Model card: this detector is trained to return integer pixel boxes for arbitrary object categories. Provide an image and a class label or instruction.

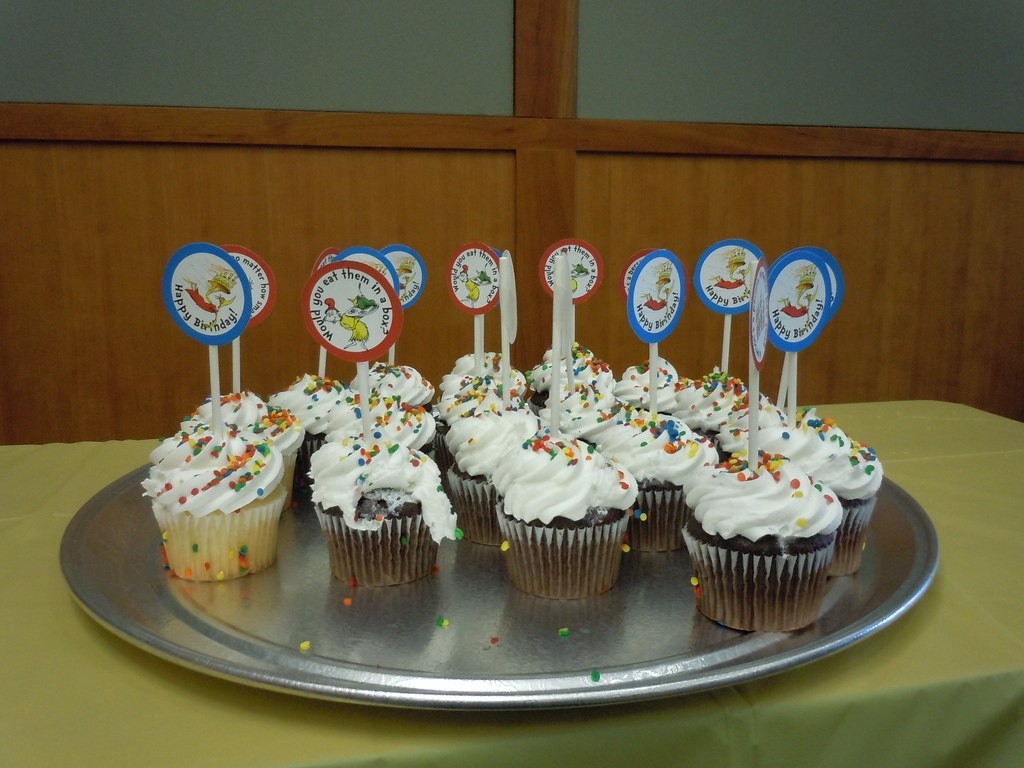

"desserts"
[139,339,884,631]
